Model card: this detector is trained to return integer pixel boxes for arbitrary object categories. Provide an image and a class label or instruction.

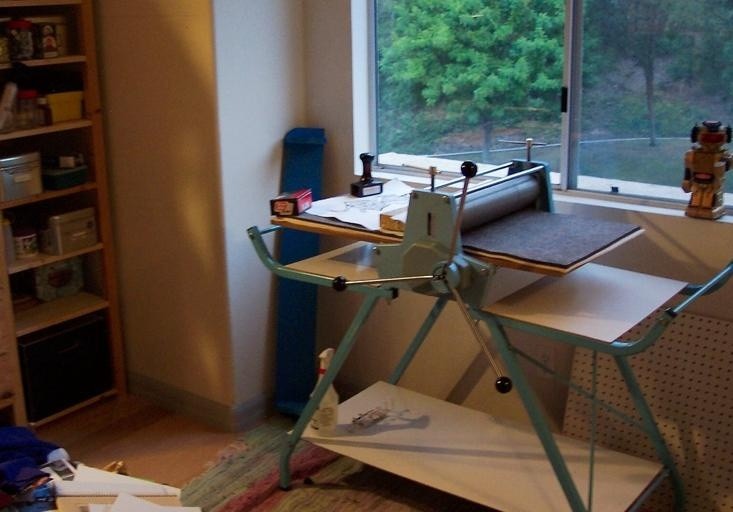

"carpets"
[178,410,490,512]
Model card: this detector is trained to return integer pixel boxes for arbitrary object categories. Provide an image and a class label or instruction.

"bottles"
[2,219,16,267]
[35,98,51,126]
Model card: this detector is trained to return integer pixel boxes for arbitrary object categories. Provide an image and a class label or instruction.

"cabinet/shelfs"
[0,0,127,429]
